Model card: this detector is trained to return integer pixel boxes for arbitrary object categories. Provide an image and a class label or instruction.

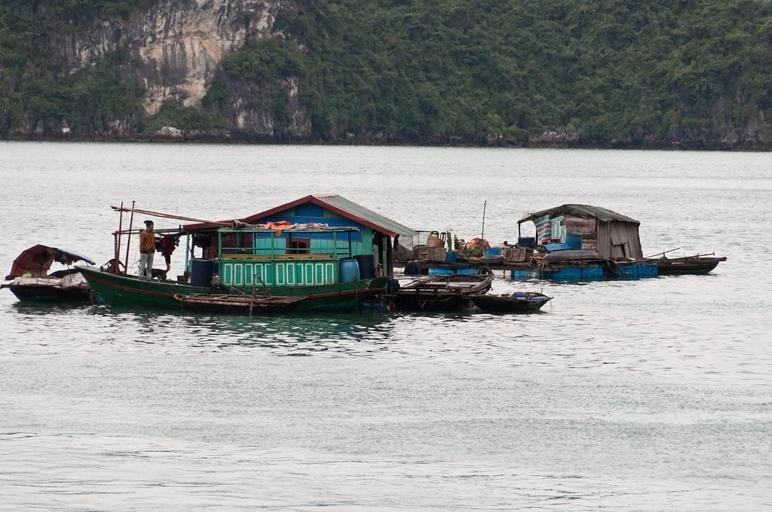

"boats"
[0,244,100,302]
[75,194,552,315]
[401,273,495,297]
[392,203,728,284]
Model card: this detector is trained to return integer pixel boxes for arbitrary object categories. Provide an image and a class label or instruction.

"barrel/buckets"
[518,237,535,248]
[191,258,214,286]
[341,258,361,284]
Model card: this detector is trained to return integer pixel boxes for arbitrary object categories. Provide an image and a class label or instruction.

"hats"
[144,220,153,226]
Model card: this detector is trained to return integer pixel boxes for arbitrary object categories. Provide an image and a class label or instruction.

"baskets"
[426,231,446,248]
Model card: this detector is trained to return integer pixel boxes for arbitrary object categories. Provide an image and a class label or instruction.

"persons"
[394,235,399,261]
[139,220,155,280]
